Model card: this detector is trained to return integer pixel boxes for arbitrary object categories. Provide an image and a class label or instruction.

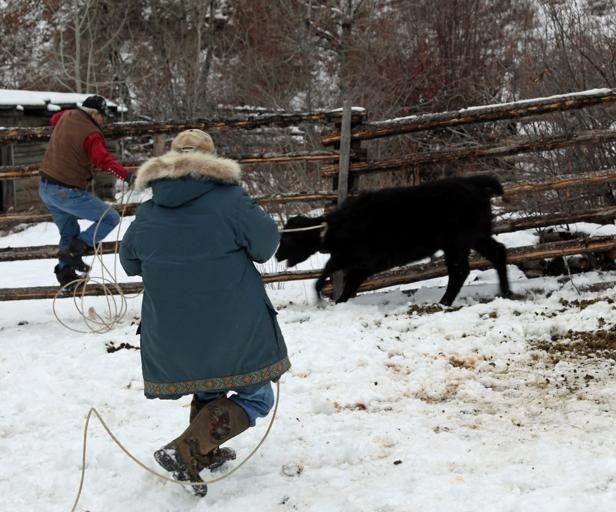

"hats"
[170,129,215,155]
[81,95,113,118]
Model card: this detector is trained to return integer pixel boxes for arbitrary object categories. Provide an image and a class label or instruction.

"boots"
[153,395,249,497]
[54,234,89,292]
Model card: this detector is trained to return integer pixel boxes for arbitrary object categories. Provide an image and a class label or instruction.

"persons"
[40,95,134,290]
[119,128,292,497]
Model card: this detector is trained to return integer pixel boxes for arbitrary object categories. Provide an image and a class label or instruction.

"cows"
[274,173,513,307]
